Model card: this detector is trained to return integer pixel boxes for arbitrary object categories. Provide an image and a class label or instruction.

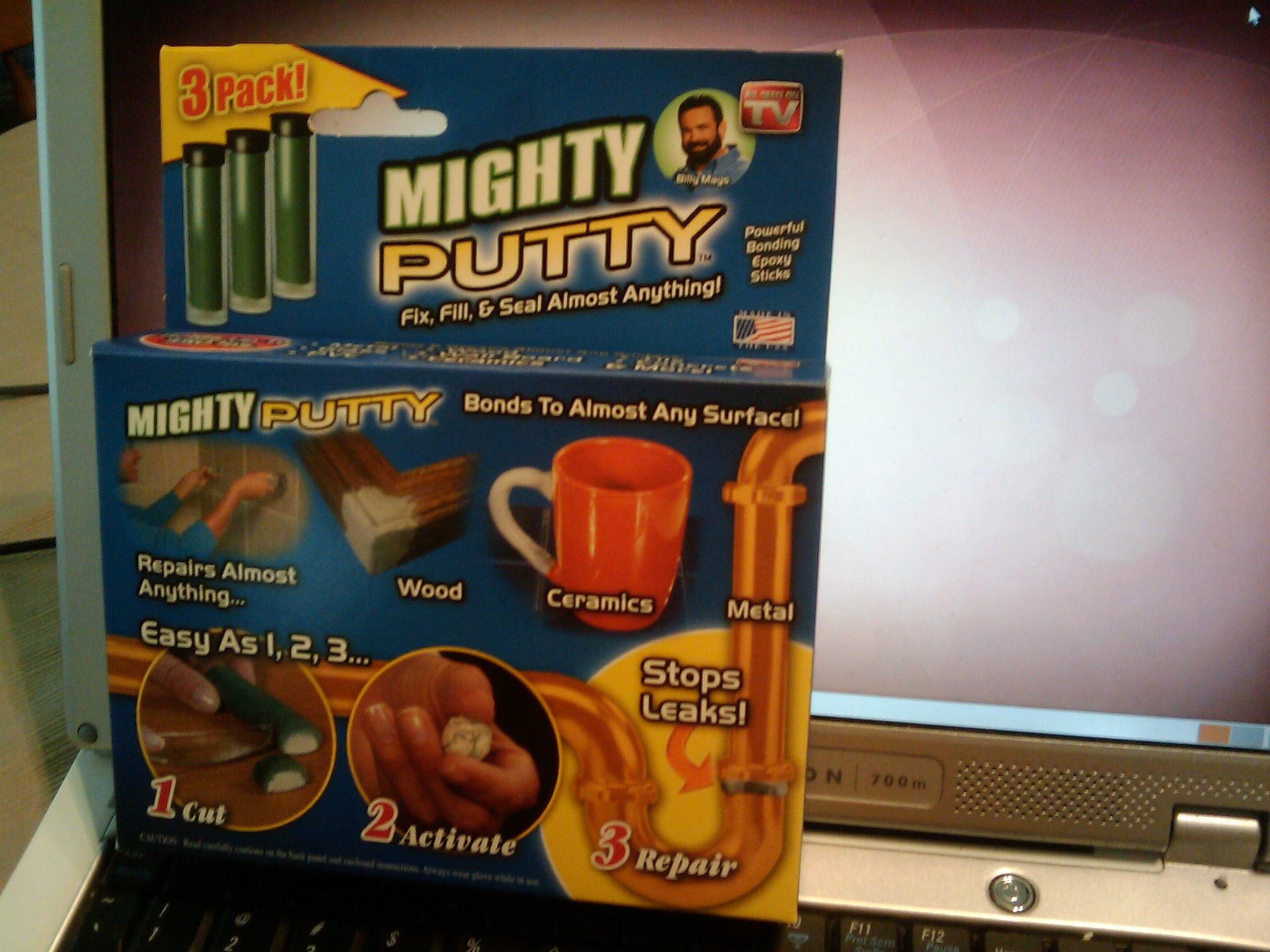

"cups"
[490,437,693,631]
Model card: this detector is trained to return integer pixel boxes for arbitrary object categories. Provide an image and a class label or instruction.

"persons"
[670,95,749,182]
[353,653,538,831]
[141,645,260,757]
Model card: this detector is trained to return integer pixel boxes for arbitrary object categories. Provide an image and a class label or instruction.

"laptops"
[0,0,1270,952]
